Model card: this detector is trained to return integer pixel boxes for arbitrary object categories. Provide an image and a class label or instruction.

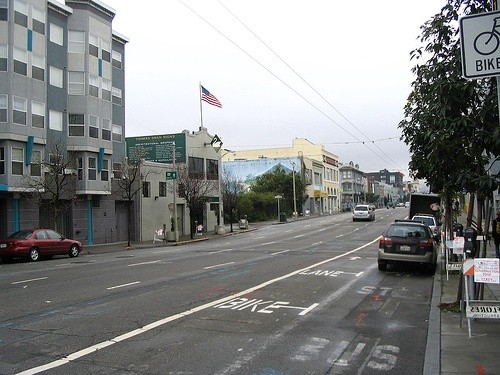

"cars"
[352,204,376,223]
[411,214,439,244]
[0,227,84,263]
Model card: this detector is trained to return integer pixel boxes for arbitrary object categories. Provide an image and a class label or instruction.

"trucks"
[409,191,441,226]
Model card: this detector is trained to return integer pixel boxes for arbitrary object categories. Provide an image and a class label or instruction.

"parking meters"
[462,227,477,318]
[453,223,464,275]
[454,200,459,223]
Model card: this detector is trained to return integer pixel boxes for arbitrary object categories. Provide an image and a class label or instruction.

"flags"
[201,86,222,108]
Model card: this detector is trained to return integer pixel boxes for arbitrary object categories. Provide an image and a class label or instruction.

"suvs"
[377,219,438,276]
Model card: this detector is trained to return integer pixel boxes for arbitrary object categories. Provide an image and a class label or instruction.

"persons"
[491,211,500,258]
[455,199,459,210]
